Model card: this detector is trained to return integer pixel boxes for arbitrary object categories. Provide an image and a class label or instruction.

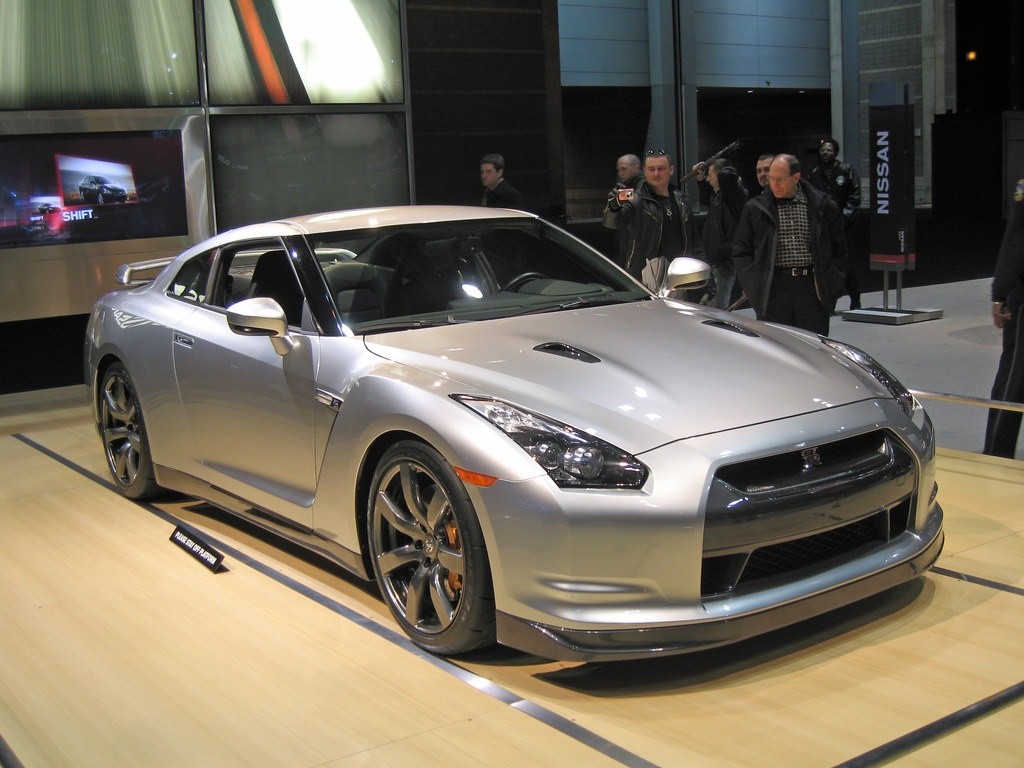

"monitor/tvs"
[0,129,188,249]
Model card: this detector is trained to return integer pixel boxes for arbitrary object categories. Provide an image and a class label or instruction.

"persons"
[400,244,467,316]
[478,153,525,293]
[983,177,1024,461]
[601,138,863,338]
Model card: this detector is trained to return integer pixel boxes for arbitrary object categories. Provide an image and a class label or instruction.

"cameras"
[618,188,634,200]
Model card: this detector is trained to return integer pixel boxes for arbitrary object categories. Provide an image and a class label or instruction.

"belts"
[773,266,816,277]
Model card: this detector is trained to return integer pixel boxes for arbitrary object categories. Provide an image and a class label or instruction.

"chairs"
[246,232,575,331]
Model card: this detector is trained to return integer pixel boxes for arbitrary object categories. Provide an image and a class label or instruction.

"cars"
[80,176,129,204]
[86,204,945,655]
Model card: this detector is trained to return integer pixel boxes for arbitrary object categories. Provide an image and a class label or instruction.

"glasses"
[645,150,668,158]
